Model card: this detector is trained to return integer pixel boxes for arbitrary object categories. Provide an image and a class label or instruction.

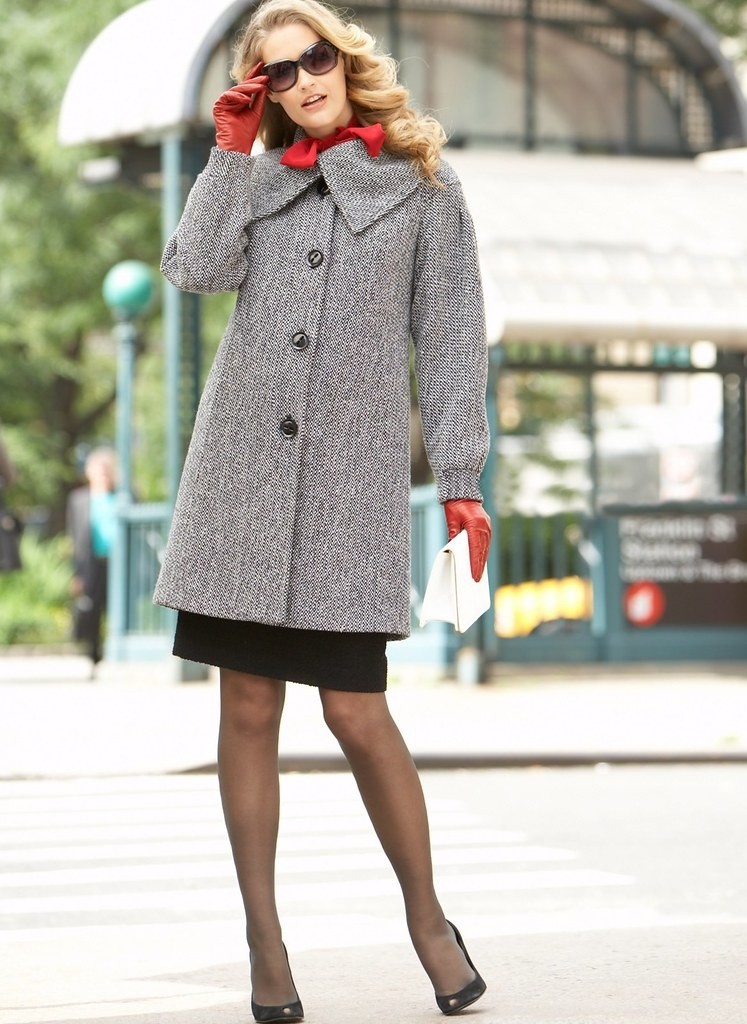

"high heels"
[250,940,304,1023]
[434,920,486,1016]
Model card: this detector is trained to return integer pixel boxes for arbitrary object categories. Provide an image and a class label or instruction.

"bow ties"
[280,113,387,169]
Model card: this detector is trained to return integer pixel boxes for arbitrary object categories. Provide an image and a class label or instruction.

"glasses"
[259,41,339,93]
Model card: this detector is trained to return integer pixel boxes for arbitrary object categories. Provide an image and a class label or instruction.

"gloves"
[213,62,269,155]
[444,499,492,582]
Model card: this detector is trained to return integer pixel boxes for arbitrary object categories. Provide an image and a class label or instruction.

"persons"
[152,0,492,1024]
[68,447,134,679]
